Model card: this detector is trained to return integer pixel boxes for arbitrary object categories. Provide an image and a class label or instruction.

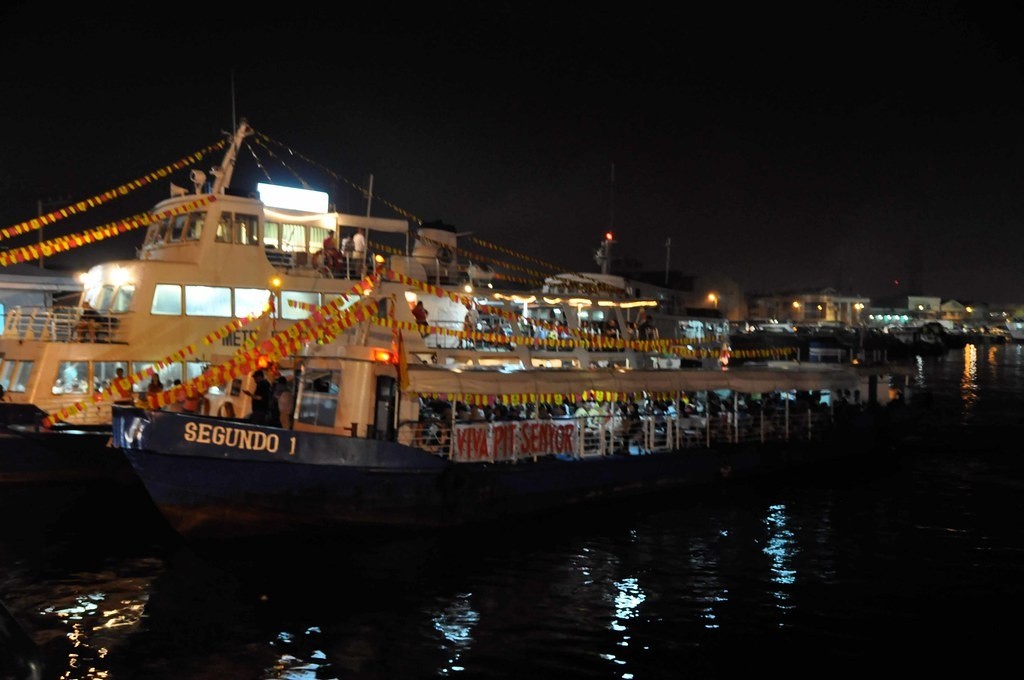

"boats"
[0,117,971,574]
[115,352,871,535]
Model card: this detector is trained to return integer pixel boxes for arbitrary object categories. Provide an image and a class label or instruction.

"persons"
[183,398,200,414]
[273,377,293,428]
[77,302,99,343]
[411,301,430,338]
[462,313,652,353]
[324,226,367,269]
[148,375,163,395]
[242,370,273,426]
[112,369,124,382]
[420,391,856,462]
